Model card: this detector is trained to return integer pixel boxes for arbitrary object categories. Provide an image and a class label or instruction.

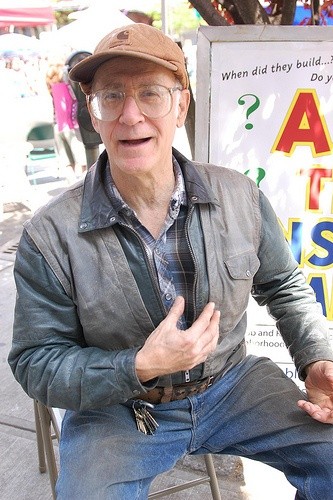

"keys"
[132,399,159,437]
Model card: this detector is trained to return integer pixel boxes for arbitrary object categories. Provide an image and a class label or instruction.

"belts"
[134,344,246,405]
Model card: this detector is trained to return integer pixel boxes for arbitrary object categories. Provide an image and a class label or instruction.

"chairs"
[34,399,221,500]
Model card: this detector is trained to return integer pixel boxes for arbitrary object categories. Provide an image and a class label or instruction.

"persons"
[47,64,86,180]
[8,23,332,500]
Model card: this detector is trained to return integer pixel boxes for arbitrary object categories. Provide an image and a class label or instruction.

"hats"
[68,23,189,89]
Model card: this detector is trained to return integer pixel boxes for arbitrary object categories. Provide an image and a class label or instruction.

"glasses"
[85,84,184,122]
